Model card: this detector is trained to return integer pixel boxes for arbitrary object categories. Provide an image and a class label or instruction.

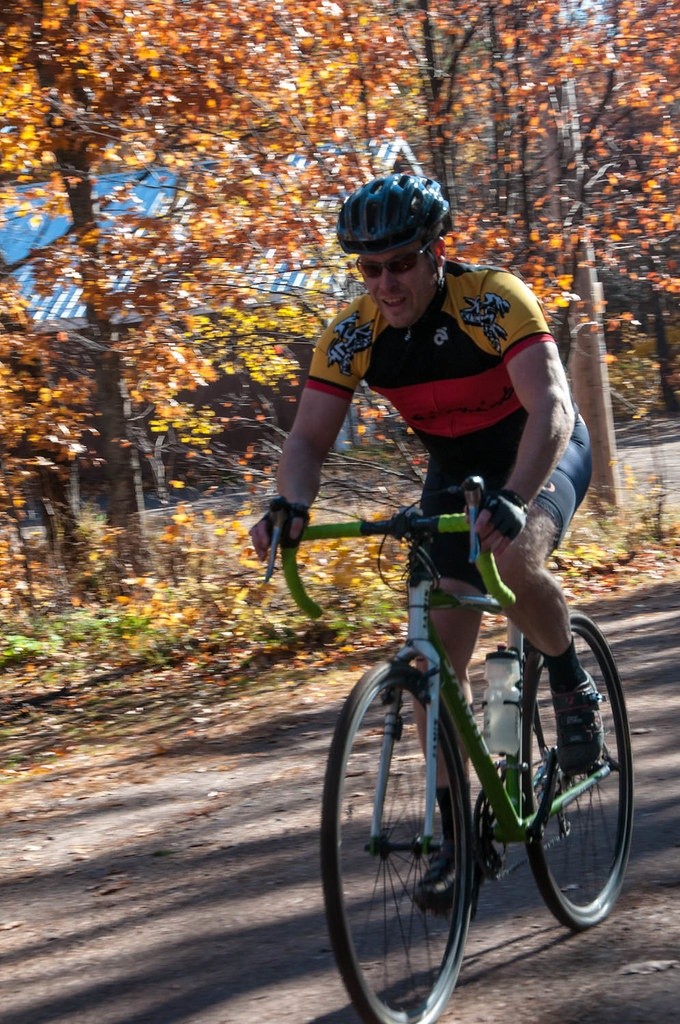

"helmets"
[335,175,451,255]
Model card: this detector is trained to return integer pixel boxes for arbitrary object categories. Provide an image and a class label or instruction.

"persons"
[251,174,604,905]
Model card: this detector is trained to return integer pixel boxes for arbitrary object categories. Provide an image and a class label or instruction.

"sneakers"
[412,834,457,907]
[552,667,608,776]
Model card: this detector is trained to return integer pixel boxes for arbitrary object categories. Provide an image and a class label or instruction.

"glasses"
[355,235,440,279]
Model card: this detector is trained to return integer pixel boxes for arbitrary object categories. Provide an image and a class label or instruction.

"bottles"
[482,643,520,755]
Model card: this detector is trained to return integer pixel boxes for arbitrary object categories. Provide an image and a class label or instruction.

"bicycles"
[265,474,633,1024]
